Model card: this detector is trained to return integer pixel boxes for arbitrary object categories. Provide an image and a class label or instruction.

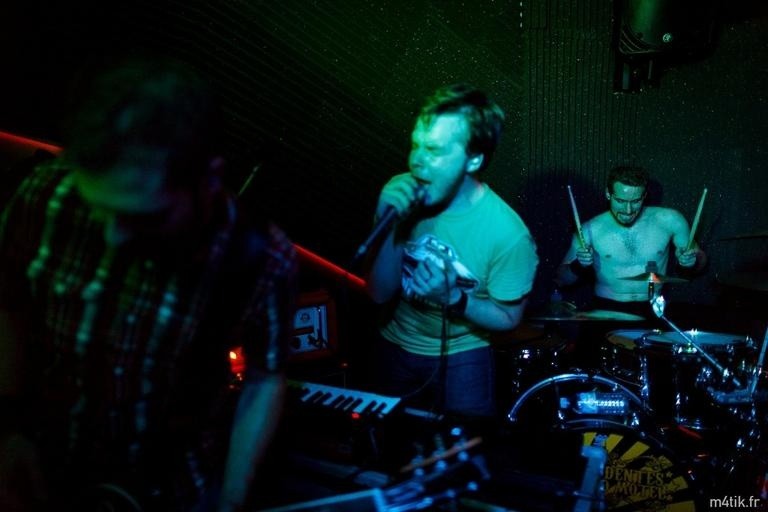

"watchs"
[443,294,468,327]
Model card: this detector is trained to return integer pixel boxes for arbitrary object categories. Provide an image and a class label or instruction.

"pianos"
[222,366,403,465]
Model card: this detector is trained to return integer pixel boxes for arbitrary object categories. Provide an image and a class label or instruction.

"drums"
[671,342,752,430]
[601,329,654,388]
[499,368,713,511]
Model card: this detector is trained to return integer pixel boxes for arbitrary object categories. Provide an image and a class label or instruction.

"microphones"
[350,182,422,263]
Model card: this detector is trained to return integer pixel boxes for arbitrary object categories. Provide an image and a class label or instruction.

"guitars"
[0,426,494,512]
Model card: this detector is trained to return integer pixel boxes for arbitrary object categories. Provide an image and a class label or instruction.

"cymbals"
[625,273,690,284]
[646,329,748,346]
[524,309,646,323]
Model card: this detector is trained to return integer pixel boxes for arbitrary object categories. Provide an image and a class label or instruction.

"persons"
[351,91,541,432]
[560,174,698,316]
